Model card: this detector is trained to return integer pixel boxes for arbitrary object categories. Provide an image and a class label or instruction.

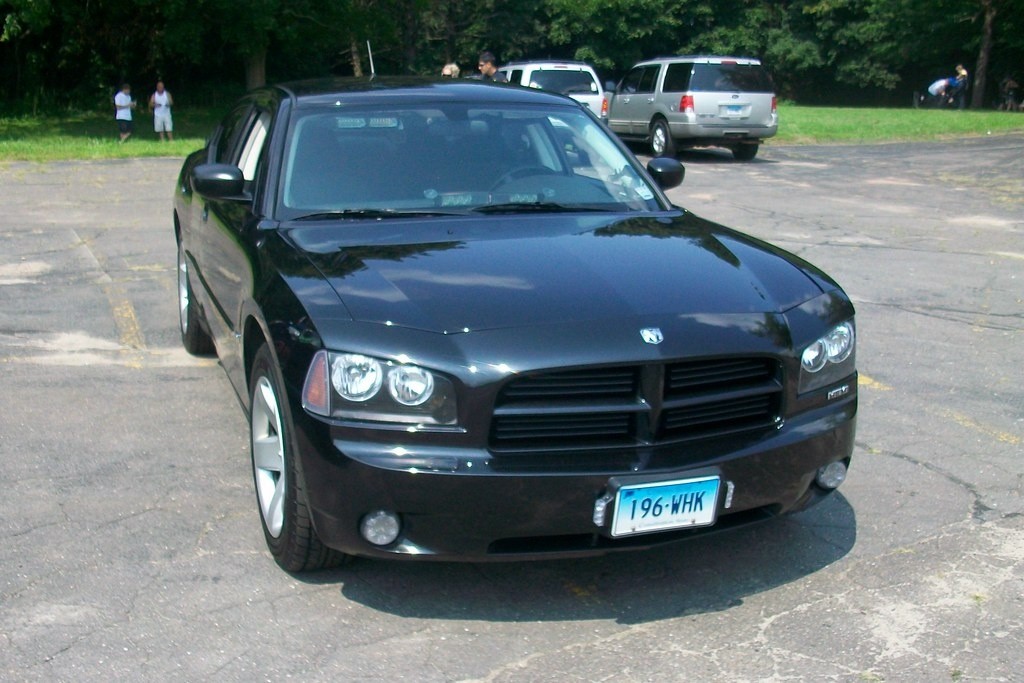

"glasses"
[478,64,487,67]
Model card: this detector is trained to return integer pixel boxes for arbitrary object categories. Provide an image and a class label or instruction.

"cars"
[496,60,608,122]
[170,72,859,574]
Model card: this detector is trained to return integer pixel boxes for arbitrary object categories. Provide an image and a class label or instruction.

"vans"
[608,54,778,161]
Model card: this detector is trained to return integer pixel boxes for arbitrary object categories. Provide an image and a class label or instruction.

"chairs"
[288,130,348,205]
[440,132,514,192]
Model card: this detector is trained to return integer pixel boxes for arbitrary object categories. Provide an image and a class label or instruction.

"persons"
[441,63,461,79]
[920,64,968,109]
[114,84,136,145]
[479,52,509,83]
[150,81,173,143]
[998,76,1024,112]
